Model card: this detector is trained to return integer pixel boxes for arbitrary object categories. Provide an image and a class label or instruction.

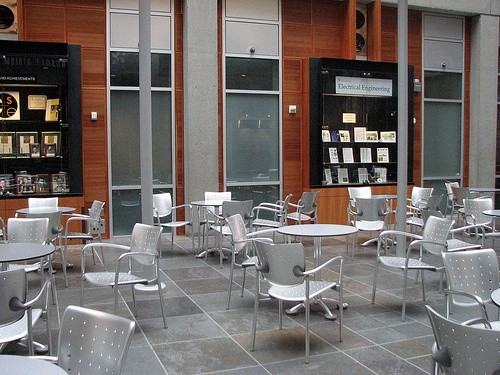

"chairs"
[348,181,500,375]
[0,191,344,375]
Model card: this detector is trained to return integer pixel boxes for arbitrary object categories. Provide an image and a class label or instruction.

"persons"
[0,146,65,196]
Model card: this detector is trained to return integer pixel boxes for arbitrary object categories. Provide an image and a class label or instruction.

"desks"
[467,187,500,239]
[0,201,359,375]
[355,193,398,247]
[481,210,500,218]
